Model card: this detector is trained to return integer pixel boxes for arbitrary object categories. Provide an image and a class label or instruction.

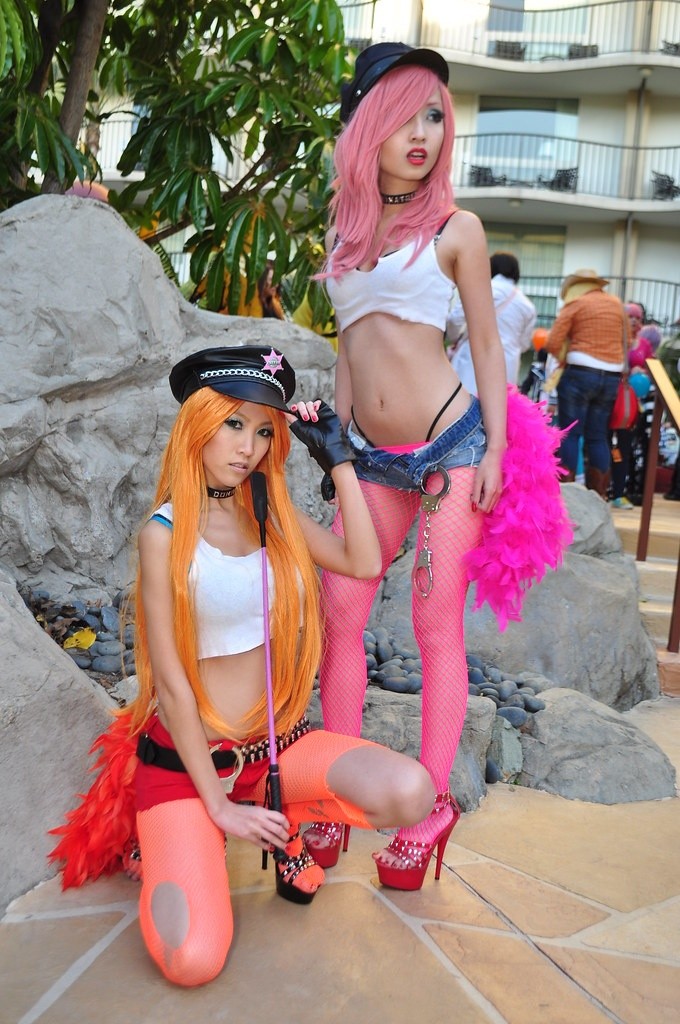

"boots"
[588,466,610,501]
[560,468,575,482]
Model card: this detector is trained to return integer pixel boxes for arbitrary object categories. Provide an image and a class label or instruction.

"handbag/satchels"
[610,379,638,429]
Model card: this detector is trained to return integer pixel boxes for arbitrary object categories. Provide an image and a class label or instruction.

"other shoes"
[612,497,634,510]
[664,490,680,501]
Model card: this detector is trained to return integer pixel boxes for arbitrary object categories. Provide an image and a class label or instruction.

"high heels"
[117,851,142,863]
[261,835,321,905]
[301,821,351,867]
[375,788,461,890]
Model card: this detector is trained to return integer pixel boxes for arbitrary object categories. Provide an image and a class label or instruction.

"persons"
[118,345,437,987]
[319,41,510,884]
[450,251,680,510]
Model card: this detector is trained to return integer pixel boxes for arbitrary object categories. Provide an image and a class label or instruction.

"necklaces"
[380,189,419,205]
[207,487,236,499]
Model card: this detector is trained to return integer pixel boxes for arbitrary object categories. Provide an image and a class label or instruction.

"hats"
[561,268,610,299]
[169,345,300,418]
[340,41,449,121]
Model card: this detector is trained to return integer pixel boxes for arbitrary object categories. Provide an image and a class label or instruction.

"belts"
[568,363,622,376]
[137,714,311,773]
